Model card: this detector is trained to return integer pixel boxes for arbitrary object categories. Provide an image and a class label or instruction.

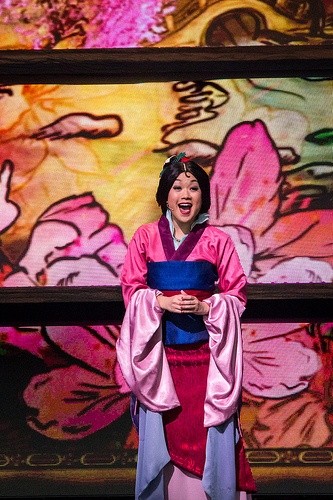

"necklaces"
[173,235,181,243]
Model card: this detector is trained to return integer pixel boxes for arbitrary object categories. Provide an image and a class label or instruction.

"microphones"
[166,201,173,211]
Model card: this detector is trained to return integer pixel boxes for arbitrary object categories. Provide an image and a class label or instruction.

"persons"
[113,155,261,500]
